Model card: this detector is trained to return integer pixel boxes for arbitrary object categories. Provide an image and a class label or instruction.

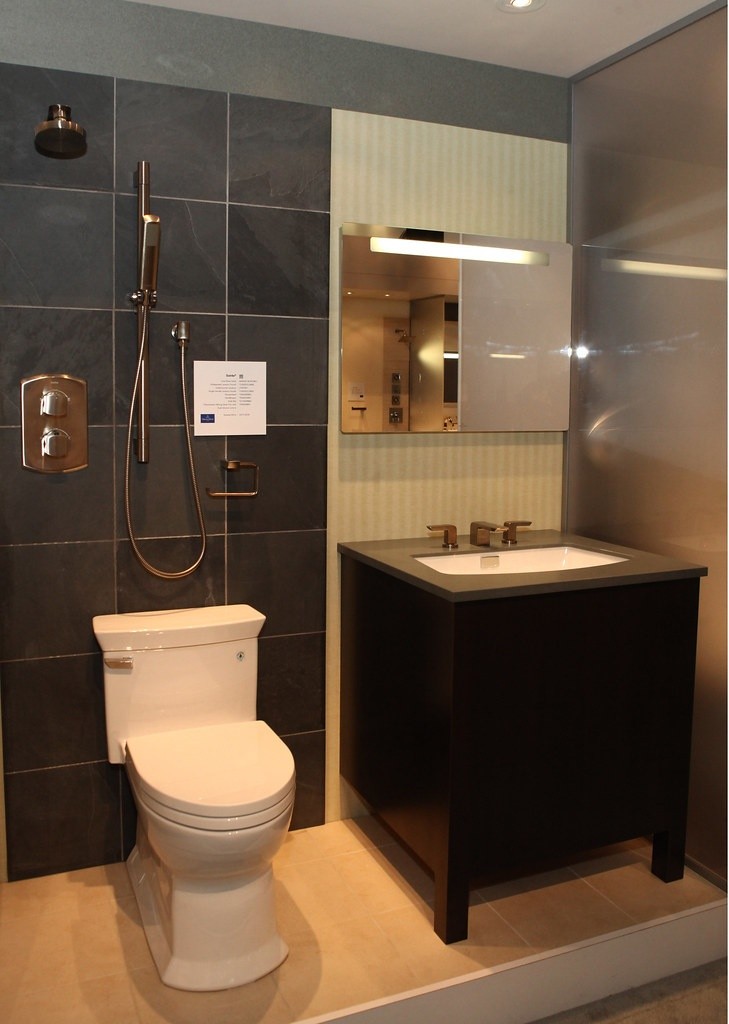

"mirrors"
[338,221,458,440]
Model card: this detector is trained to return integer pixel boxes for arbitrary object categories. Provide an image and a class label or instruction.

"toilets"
[91,600,298,994]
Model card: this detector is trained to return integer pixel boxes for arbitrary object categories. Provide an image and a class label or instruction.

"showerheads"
[135,212,163,309]
[31,102,89,161]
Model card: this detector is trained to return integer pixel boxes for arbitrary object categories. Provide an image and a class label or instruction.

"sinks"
[409,539,638,578]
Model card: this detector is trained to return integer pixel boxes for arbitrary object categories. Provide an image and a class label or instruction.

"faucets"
[469,520,509,548]
